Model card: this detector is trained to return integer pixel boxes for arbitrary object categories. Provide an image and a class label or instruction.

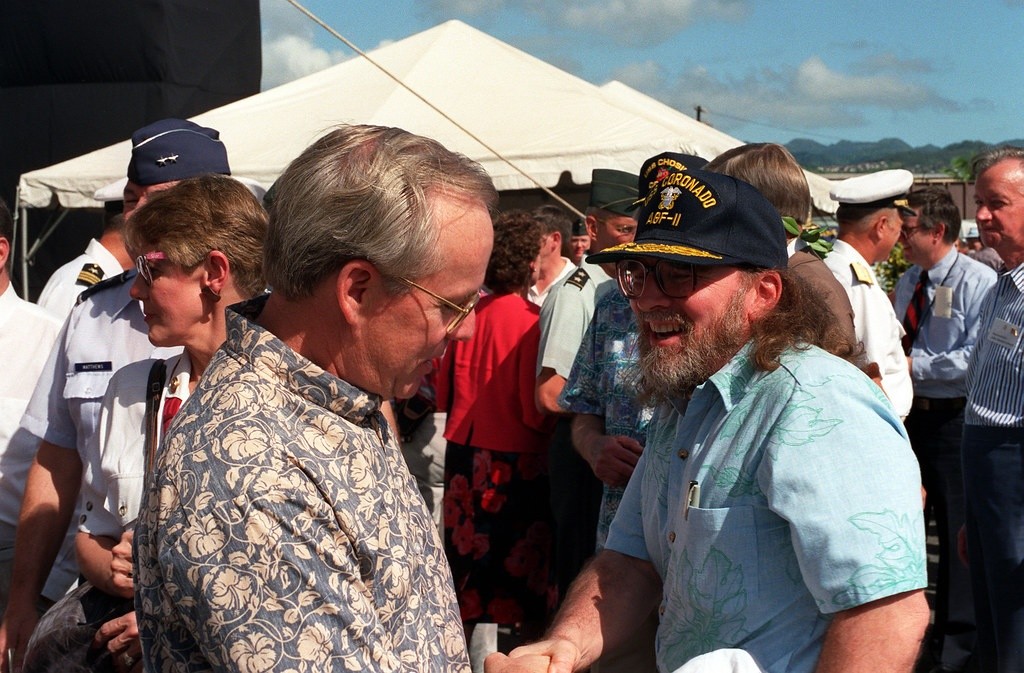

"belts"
[913,396,968,410]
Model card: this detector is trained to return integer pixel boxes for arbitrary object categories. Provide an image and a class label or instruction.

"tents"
[18,19,840,302]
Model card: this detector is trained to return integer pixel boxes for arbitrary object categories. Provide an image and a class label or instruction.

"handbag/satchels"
[21,577,120,673]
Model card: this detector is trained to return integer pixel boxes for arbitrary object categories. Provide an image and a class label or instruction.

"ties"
[900,269,930,351]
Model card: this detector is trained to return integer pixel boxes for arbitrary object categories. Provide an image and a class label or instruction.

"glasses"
[329,252,482,336]
[135,248,172,287]
[615,258,697,300]
[595,214,639,236]
[900,223,939,237]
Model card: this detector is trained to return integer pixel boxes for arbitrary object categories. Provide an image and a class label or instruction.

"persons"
[382,169,656,652]
[823,169,914,422]
[964,226,1004,272]
[0,199,79,603]
[36,174,136,316]
[509,171,931,673]
[891,186,998,673]
[75,172,270,673]
[958,144,1024,673]
[139,124,551,673]
[0,119,233,673]
[701,142,857,365]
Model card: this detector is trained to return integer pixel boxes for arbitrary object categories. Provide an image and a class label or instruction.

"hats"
[589,167,645,220]
[636,152,710,208]
[127,119,231,186]
[584,169,788,278]
[828,168,918,220]
